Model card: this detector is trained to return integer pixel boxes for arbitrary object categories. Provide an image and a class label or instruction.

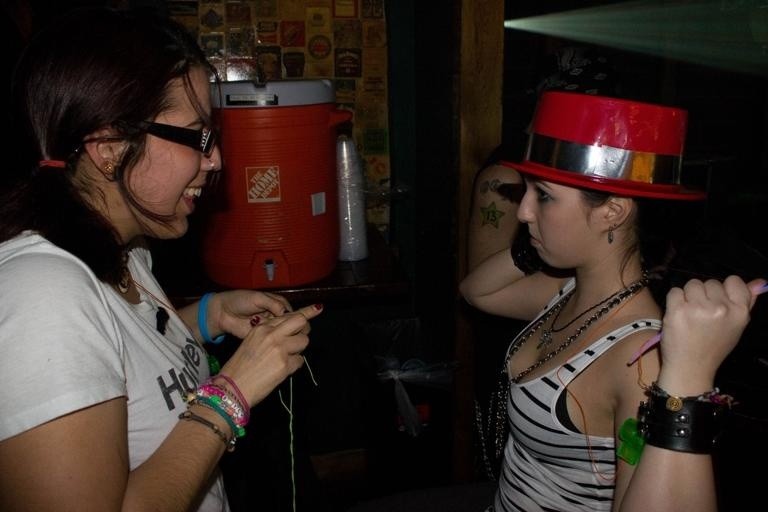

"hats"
[493,87,710,202]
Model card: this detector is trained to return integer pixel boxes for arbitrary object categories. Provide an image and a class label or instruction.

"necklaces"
[484,270,654,461]
[118,252,132,293]
[535,289,623,350]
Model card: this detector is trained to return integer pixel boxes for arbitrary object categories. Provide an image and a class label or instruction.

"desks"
[155,223,411,507]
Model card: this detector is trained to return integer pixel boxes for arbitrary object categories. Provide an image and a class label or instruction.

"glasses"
[114,116,220,159]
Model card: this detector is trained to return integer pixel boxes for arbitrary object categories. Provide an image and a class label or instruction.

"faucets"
[262,255,278,282]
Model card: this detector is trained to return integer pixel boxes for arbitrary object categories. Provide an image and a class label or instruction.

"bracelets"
[176,371,253,453]
[511,236,541,276]
[198,290,227,346]
[636,381,737,455]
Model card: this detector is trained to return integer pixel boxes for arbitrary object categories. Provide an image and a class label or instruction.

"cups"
[336,134,370,263]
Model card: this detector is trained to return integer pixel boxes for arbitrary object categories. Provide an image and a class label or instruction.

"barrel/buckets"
[193,76,340,290]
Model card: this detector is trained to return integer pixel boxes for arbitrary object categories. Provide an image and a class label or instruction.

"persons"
[0,9,326,512]
[464,140,525,483]
[455,91,767,511]
